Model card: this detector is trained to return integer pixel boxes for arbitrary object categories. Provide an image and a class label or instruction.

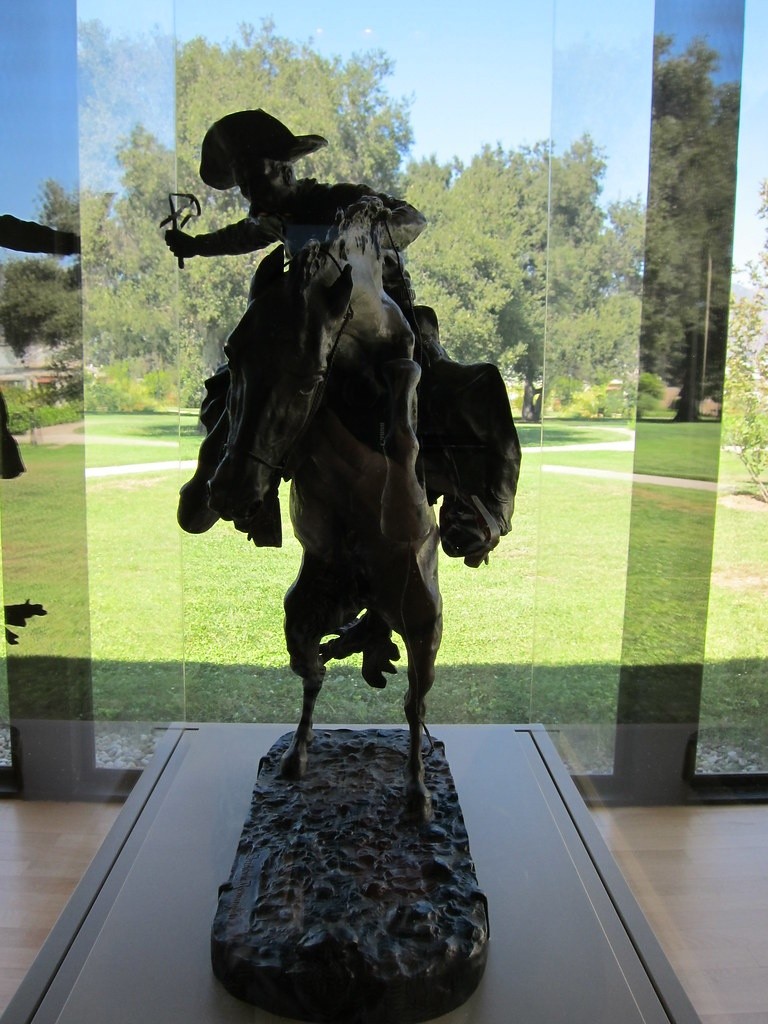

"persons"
[159,109,515,557]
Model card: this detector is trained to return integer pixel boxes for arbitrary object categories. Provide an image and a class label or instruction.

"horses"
[204,244,444,824]
